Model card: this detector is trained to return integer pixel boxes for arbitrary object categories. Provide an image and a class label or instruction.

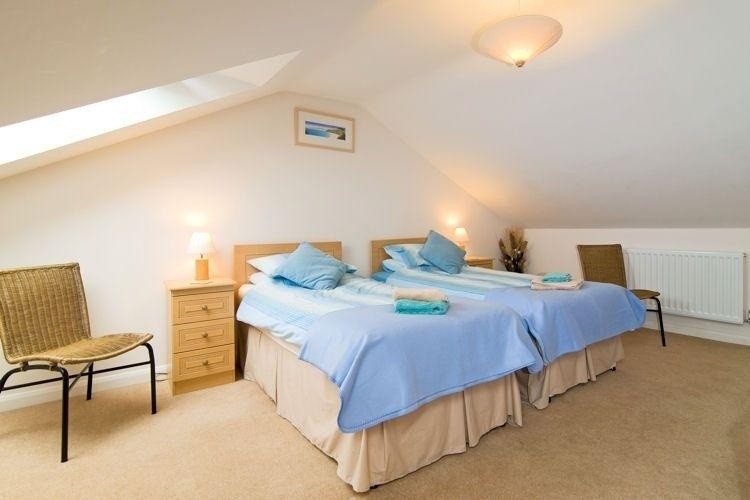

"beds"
[228,237,548,494]
[369,233,651,414]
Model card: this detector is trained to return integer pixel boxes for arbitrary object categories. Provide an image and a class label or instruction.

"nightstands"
[460,253,496,271]
[163,272,240,400]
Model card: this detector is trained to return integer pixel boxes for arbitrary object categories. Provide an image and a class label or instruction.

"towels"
[541,270,573,284]
[392,283,451,300]
[395,298,454,317]
[528,273,587,294]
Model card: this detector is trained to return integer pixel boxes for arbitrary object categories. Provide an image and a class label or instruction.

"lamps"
[470,12,567,73]
[186,228,216,284]
[450,222,471,256]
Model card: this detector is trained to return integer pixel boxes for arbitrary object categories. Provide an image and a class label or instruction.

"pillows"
[243,228,470,292]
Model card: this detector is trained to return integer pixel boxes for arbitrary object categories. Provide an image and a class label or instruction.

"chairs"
[574,242,670,351]
[1,259,160,468]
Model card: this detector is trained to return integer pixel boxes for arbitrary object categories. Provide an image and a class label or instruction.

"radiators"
[614,244,750,328]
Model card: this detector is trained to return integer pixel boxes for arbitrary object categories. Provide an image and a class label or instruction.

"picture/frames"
[292,104,358,157]
